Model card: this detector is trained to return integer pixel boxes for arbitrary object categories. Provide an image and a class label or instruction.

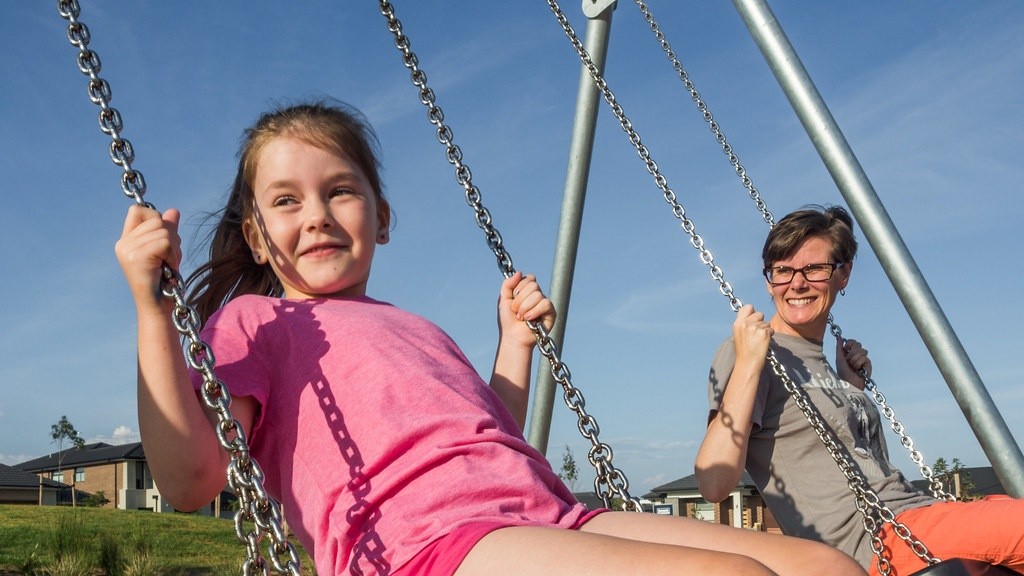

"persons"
[696,205,1024,576]
[115,102,869,576]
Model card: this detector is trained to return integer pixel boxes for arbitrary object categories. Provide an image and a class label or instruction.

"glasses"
[763,262,844,284]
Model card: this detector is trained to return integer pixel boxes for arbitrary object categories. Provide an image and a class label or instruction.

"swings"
[542,0,1024,576]
[52,0,648,576]
